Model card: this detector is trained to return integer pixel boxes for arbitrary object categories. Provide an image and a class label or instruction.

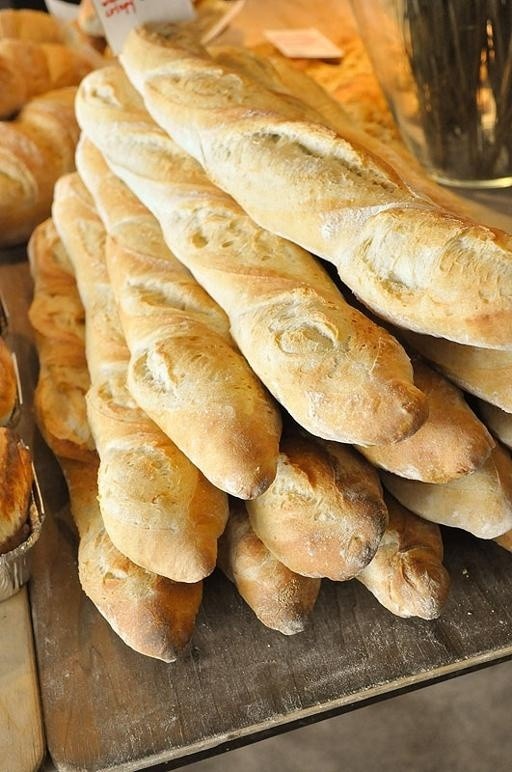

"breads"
[1,0,512,663]
